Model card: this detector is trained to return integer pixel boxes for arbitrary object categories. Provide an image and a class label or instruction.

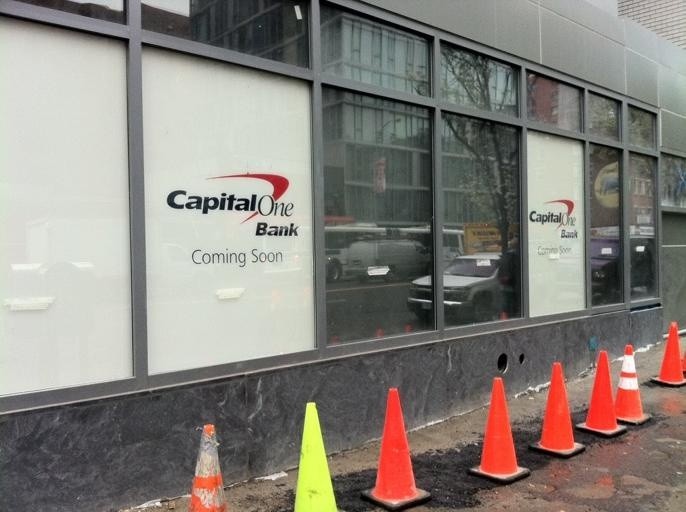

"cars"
[405,251,520,323]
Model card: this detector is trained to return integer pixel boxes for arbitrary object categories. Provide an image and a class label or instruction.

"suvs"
[590,235,656,305]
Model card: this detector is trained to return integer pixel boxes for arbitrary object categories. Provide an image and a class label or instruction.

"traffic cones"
[574,351,627,440]
[188,424,227,512]
[330,335,338,345]
[293,401,339,512]
[650,322,686,388]
[499,312,508,320]
[615,344,652,426]
[360,387,432,512]
[465,377,530,485]
[376,329,384,337]
[403,324,413,333]
[528,362,585,460]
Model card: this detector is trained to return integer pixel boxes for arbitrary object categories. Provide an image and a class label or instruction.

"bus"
[324,223,465,283]
